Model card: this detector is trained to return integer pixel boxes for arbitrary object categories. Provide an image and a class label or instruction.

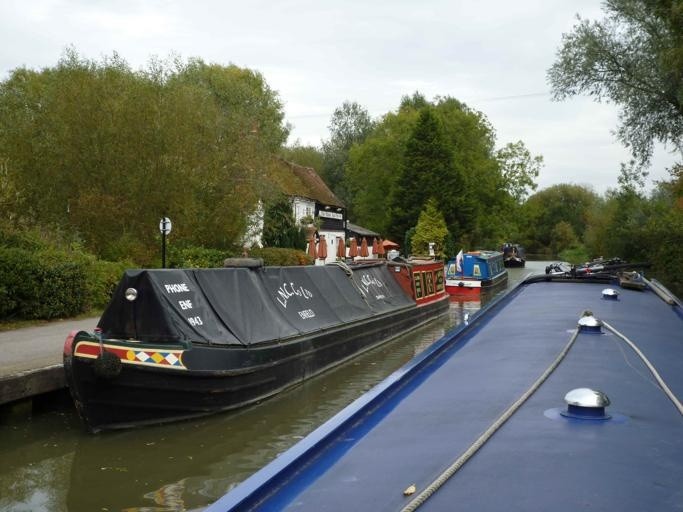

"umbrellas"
[347,239,358,262]
[334,239,345,261]
[380,239,399,249]
[377,238,386,259]
[316,234,328,265]
[306,237,318,262]
[358,238,369,260]
[371,238,378,259]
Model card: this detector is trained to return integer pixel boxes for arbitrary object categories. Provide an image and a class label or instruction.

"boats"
[503,243,525,267]
[445,250,508,295]
[196,258,683,507]
[64,258,450,439]
